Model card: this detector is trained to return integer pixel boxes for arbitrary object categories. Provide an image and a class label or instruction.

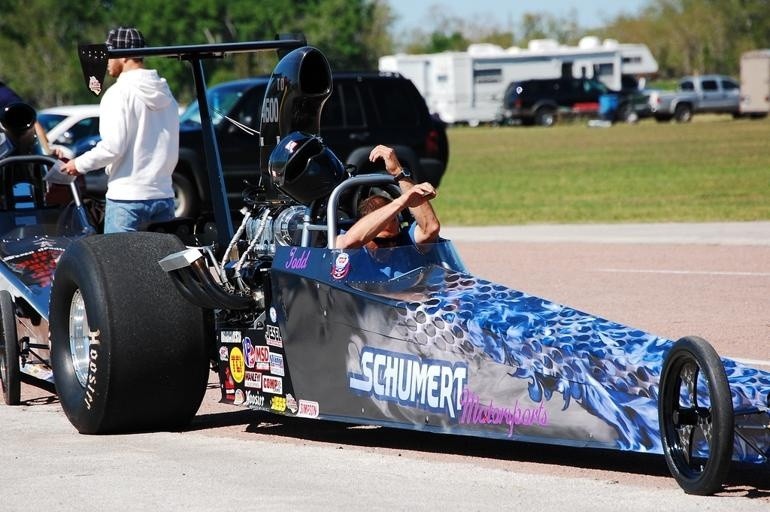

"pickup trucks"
[646,75,741,124]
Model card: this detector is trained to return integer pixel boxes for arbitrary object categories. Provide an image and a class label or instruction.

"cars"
[598,88,657,124]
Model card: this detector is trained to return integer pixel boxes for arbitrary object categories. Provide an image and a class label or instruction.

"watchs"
[394,168,411,180]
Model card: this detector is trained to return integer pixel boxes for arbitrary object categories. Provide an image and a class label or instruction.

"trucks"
[739,50,770,114]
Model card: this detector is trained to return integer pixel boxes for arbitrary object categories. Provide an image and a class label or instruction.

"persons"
[60,24,180,235]
[336,143,441,280]
[0,82,49,153]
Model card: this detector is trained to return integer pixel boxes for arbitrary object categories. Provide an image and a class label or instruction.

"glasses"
[372,224,406,247]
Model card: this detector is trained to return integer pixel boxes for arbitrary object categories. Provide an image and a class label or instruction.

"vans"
[499,77,620,127]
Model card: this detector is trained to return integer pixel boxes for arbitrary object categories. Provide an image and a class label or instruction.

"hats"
[105,26,146,49]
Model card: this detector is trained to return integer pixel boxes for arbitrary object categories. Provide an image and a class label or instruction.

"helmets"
[0,103,37,134]
[267,130,347,204]
